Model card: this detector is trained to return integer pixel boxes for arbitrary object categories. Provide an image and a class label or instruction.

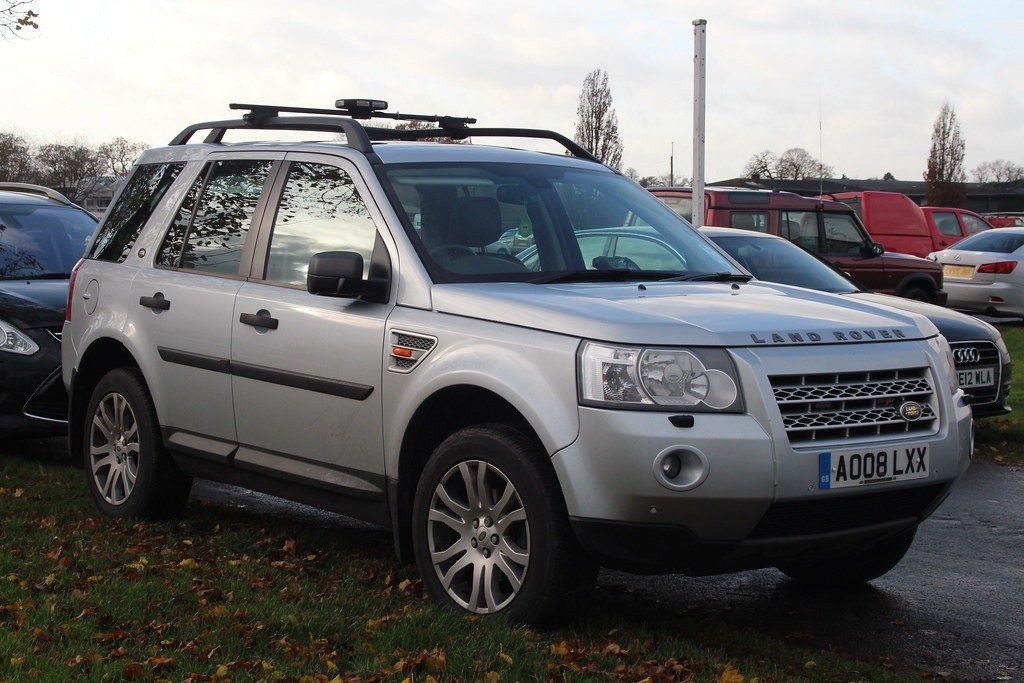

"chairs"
[449,195,529,270]
[939,220,959,235]
[734,213,756,231]
[780,219,802,248]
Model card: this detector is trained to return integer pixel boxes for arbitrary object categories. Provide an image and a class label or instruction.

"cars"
[408,185,1024,422]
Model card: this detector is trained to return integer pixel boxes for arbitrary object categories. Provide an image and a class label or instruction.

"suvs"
[62,96,980,629]
[0,181,103,447]
